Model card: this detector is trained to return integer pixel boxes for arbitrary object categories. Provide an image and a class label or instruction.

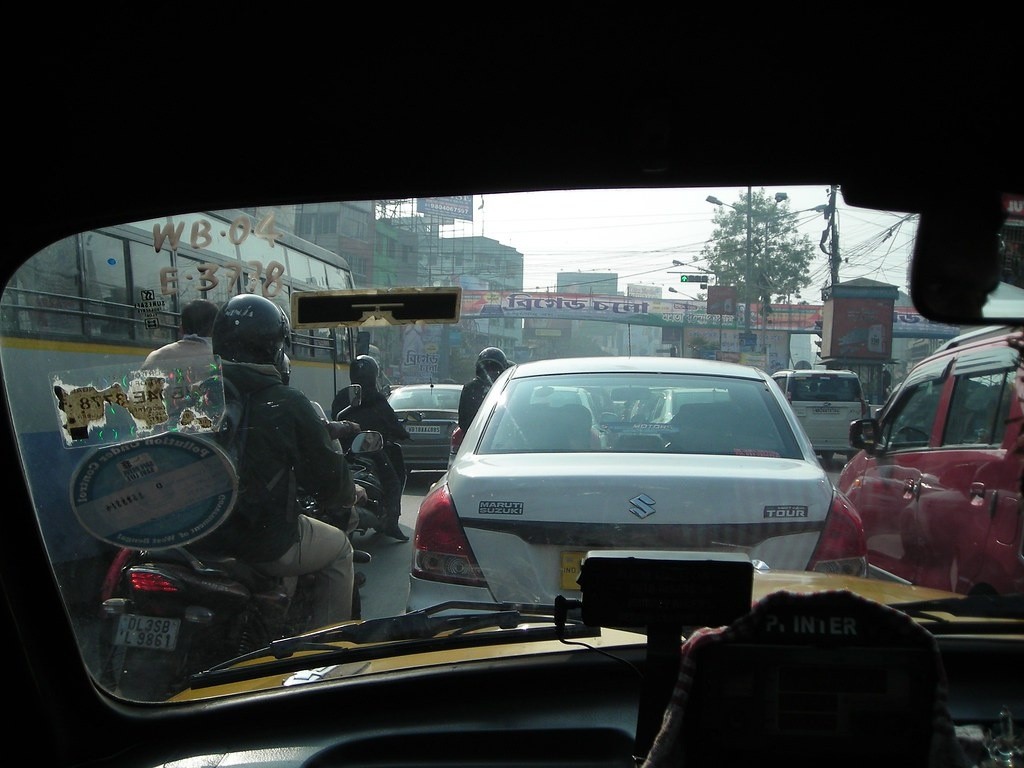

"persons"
[127,295,519,631]
[810,382,819,392]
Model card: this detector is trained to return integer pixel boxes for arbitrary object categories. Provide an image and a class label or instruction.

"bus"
[0,207,370,569]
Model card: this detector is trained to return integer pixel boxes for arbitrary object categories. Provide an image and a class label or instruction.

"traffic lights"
[700,284,708,289]
[681,275,709,284]
[814,318,825,359]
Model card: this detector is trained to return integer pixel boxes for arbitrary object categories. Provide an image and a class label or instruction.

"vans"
[772,371,867,467]
[835,320,1022,599]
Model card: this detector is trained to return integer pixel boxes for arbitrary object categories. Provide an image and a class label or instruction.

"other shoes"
[384,525,411,542]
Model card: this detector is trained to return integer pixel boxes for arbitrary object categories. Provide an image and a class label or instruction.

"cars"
[385,380,464,476]
[406,354,869,622]
[531,384,731,449]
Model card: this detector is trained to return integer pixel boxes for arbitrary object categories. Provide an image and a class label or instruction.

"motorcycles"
[98,381,423,703]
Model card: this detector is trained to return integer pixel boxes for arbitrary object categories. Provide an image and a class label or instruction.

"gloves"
[400,430,410,441]
[342,484,367,508]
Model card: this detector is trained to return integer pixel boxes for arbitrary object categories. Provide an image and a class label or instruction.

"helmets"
[350,355,379,380]
[476,348,508,371]
[211,294,292,358]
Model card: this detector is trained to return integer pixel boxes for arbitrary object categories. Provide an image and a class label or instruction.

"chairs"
[525,402,599,451]
[674,403,747,449]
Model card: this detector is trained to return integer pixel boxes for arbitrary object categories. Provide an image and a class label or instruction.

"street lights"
[672,258,723,352]
[704,192,789,371]
